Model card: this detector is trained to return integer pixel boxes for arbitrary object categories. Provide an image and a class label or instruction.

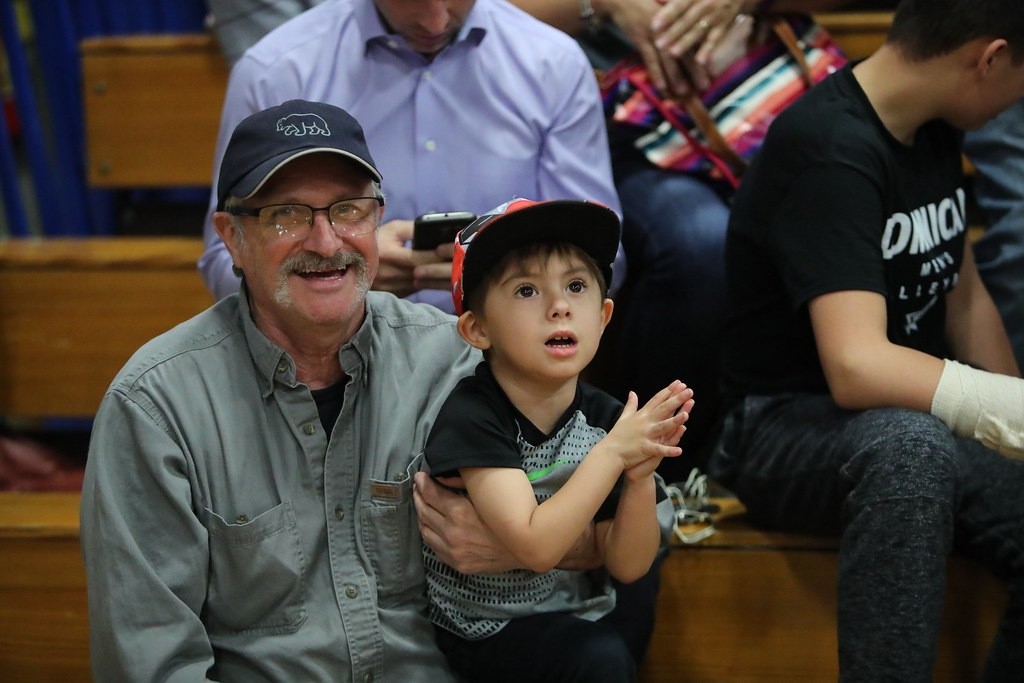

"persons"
[422,197,695,683]
[199,0,1024,683]
[80,99,598,683]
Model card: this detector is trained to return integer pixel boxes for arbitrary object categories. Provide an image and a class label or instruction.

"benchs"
[0,38,1007,683]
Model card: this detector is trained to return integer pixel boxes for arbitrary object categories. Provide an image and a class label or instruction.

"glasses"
[226,197,384,246]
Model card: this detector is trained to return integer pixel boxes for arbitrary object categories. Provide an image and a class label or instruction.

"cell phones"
[412,211,477,251]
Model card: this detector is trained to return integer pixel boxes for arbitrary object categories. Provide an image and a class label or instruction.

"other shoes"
[673,469,748,525]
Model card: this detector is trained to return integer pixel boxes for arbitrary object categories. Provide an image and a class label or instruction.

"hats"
[215,98,383,213]
[452,197,622,317]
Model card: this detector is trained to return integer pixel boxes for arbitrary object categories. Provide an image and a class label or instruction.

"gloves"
[933,359,1024,460]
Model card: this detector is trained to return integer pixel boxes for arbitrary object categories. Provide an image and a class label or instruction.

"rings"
[697,19,711,30]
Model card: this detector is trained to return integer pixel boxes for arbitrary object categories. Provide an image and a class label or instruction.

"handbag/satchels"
[603,4,849,186]
